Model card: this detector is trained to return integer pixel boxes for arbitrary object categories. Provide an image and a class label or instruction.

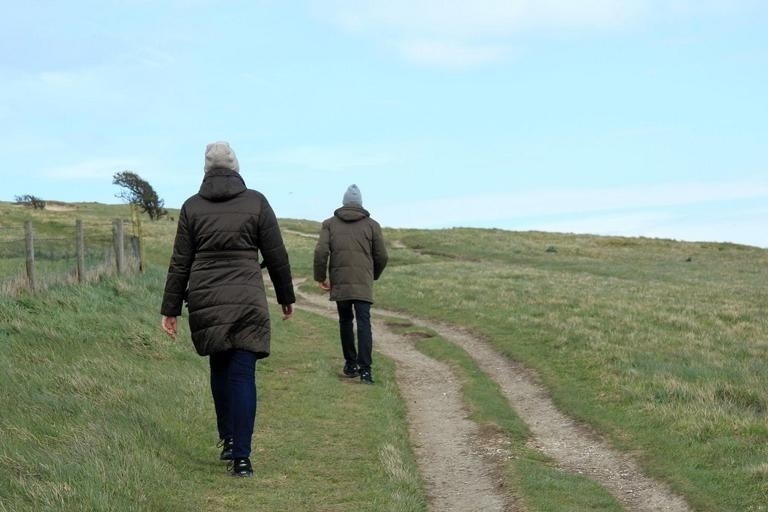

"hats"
[343,184,362,206]
[204,141,240,173]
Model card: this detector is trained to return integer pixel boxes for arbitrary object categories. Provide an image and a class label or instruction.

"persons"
[312,183,388,383]
[160,140,295,478]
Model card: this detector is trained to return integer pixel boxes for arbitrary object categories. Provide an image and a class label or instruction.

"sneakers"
[216,436,254,477]
[343,362,375,384]
